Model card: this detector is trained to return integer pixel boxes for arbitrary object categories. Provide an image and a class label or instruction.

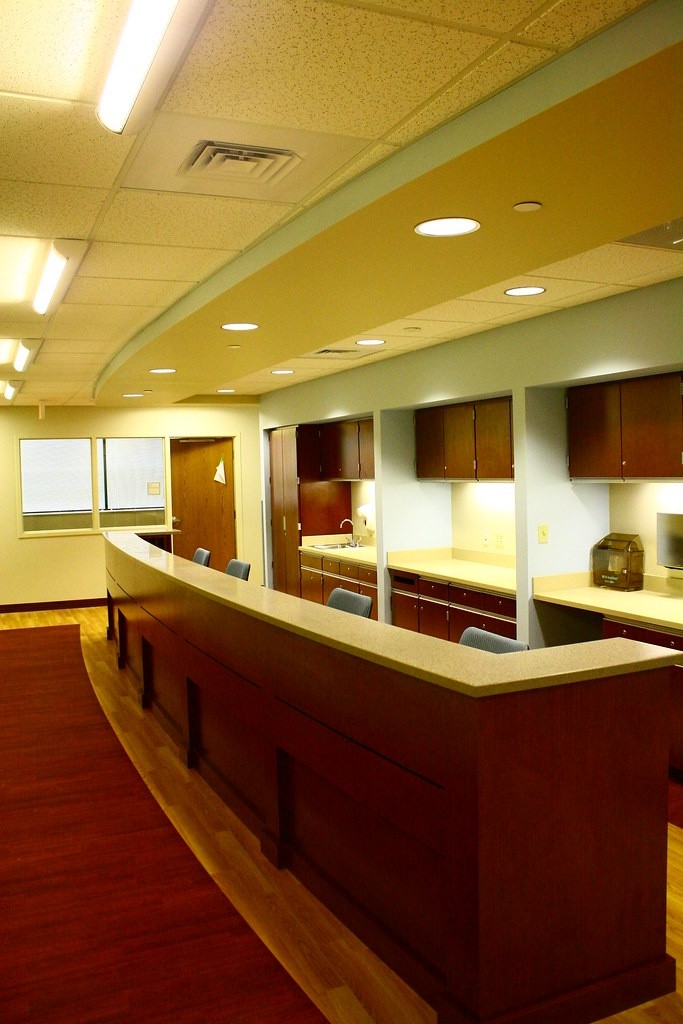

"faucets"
[340,519,356,545]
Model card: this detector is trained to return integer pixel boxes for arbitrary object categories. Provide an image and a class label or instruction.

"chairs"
[458,625,529,655]
[324,588,374,620]
[224,558,251,581]
[192,547,211,567]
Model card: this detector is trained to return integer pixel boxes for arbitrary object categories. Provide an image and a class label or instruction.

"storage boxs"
[591,531,646,592]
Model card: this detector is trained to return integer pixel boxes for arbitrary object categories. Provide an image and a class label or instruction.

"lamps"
[94,0,208,136]
[4,380,22,400]
[32,238,88,317]
[13,338,39,373]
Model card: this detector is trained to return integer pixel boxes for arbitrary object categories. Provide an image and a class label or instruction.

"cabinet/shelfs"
[316,418,375,484]
[563,370,683,482]
[298,551,516,645]
[413,396,515,483]
[266,427,351,598]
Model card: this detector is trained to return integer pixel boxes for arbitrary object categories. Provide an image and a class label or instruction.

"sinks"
[316,542,362,549]
